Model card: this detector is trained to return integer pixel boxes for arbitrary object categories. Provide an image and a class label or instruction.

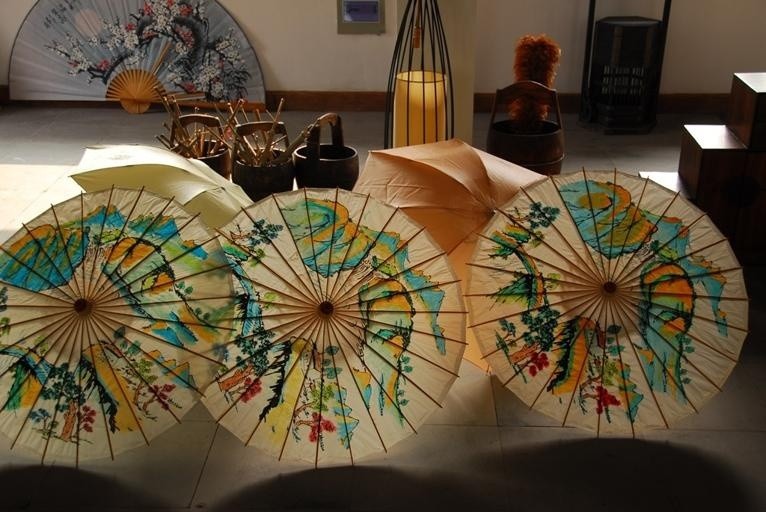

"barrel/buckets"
[293,109,360,192]
[232,122,293,204]
[169,112,231,182]
[485,78,565,177]
[590,17,663,127]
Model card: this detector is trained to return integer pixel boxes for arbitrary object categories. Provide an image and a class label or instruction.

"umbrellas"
[354,138,547,259]
[181,185,469,469]
[0,181,235,465]
[70,142,254,230]
[467,168,752,437]
